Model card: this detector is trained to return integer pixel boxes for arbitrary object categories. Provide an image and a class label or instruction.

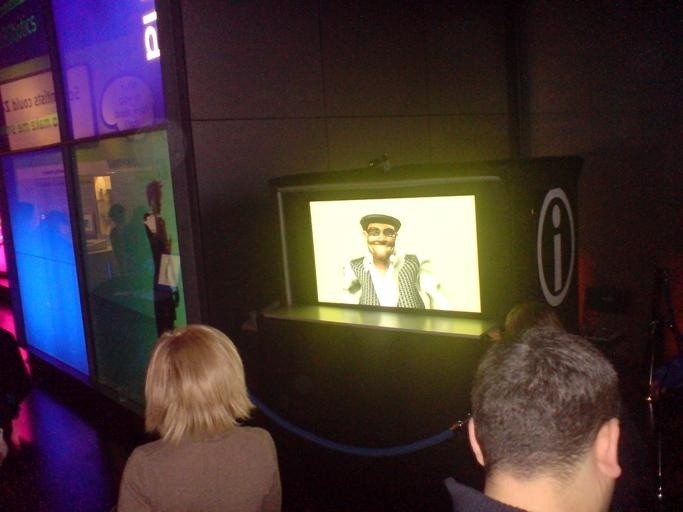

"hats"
[360,214,401,233]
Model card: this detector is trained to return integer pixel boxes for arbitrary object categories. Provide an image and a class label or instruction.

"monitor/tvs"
[301,185,487,318]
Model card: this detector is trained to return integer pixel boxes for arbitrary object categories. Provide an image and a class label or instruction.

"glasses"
[367,226,396,237]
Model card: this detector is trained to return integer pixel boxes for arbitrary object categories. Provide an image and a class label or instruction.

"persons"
[113,325,284,512]
[139,181,178,335]
[505,300,569,341]
[106,204,131,279]
[0,328,30,436]
[342,212,438,310]
[446,326,623,512]
